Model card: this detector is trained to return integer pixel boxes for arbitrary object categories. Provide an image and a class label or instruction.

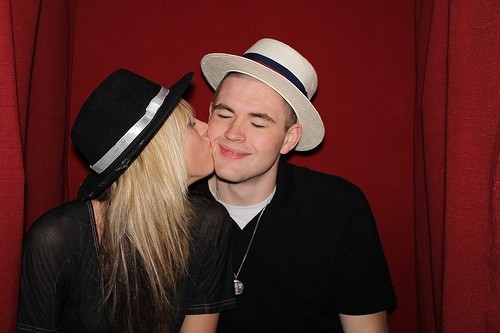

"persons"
[14,67,233,333]
[189,38,399,333]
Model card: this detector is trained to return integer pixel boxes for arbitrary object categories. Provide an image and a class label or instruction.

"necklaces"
[233,192,273,295]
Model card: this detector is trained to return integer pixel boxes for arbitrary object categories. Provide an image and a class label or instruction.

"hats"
[71,69,194,201]
[200,38,325,151]
